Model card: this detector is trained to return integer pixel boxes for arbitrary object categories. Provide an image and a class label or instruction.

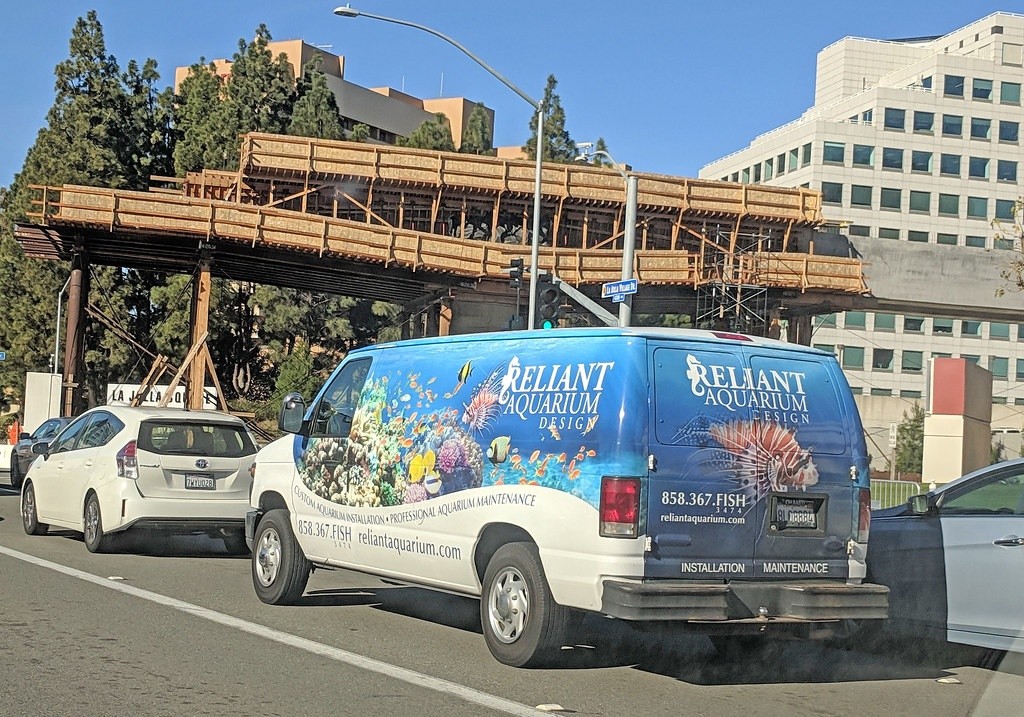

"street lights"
[573,147,641,325]
[334,2,547,327]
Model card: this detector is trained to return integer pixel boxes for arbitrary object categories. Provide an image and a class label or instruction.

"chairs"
[191,432,214,456]
[161,432,186,454]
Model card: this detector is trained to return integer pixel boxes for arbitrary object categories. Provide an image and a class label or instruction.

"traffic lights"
[536,281,559,329]
[508,258,523,288]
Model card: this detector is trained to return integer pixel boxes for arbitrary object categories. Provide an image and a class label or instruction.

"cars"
[822,458,1024,657]
[18,404,259,556]
[9,417,77,488]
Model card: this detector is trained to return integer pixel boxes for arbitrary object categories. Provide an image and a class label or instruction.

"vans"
[244,326,890,671]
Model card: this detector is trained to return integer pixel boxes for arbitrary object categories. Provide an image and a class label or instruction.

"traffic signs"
[601,280,637,302]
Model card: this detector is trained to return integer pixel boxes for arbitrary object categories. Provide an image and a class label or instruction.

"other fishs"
[672,404,819,532]
[381,359,598,495]
